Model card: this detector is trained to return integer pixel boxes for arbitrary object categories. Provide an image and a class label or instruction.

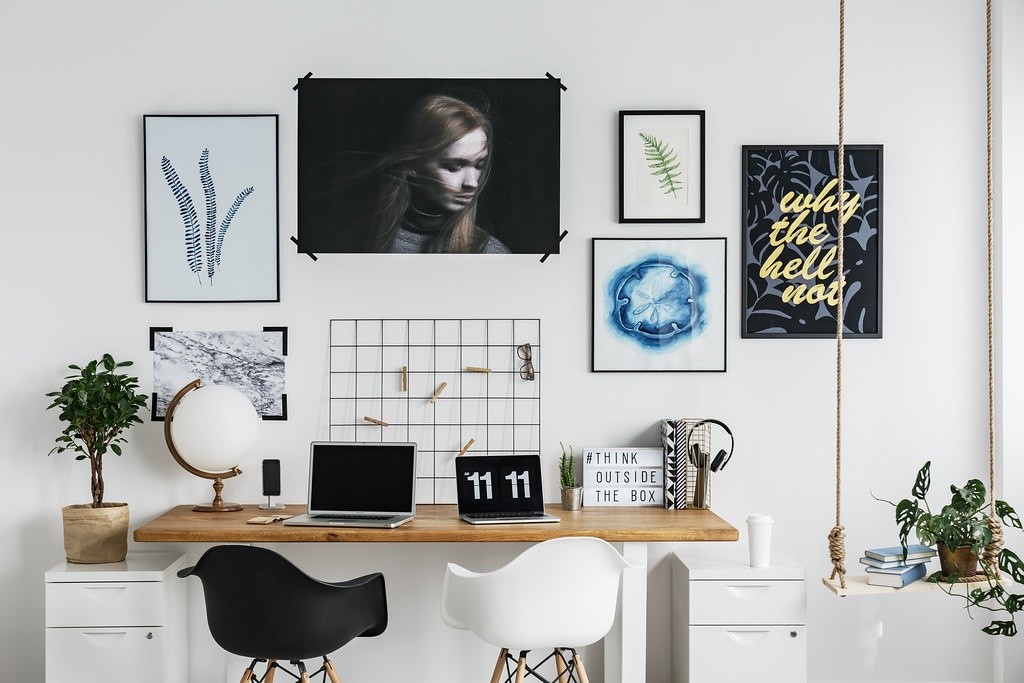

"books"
[859,557,931,569]
[866,563,927,588]
[247,514,292,525]
[865,564,919,575]
[865,545,938,563]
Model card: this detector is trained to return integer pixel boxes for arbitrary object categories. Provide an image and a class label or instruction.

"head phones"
[688,419,734,472]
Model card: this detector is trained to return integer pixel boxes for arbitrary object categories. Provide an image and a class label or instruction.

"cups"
[747,513,773,567]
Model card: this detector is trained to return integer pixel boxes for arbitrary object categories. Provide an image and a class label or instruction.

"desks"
[133,503,739,683]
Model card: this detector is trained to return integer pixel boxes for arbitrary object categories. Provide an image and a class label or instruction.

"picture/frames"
[591,237,727,373]
[741,144,884,339]
[143,114,279,303]
[618,109,706,224]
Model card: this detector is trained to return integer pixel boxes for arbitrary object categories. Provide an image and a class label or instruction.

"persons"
[307,95,512,254]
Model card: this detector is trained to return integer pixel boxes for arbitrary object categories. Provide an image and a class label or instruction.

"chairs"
[177,545,389,683]
[439,537,631,683]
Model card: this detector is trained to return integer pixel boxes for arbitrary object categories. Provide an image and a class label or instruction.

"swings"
[824,0,1012,598]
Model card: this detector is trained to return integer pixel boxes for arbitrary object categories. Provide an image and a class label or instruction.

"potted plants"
[873,461,1024,638]
[557,442,585,511]
[44,353,150,565]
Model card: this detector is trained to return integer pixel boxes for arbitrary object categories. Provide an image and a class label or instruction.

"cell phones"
[263,459,280,496]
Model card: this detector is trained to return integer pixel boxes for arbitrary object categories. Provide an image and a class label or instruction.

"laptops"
[283,442,418,528]
[455,454,561,524]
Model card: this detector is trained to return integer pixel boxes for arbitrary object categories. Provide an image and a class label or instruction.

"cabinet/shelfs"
[671,550,807,683]
[44,551,188,683]
